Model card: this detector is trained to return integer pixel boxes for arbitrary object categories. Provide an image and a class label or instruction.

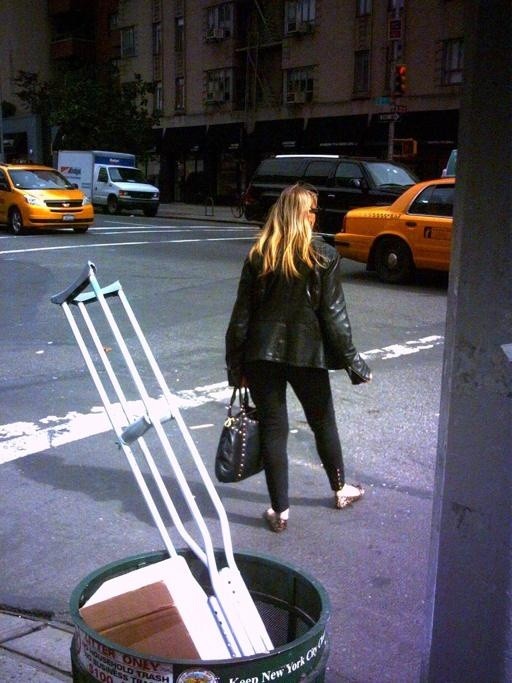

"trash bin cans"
[69,546,331,683]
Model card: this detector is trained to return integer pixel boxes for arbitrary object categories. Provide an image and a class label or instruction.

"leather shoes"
[334,484,365,510]
[264,508,287,533]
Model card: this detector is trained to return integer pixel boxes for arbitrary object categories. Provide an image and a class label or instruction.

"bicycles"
[231,192,246,218]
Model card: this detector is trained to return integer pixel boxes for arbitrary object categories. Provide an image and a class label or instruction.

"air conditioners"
[287,21,308,33]
[204,90,222,101]
[287,92,306,103]
[205,29,224,39]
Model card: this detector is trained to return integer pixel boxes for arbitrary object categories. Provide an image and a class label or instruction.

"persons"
[224,181,372,534]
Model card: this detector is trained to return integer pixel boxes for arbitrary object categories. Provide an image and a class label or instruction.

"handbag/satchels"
[215,407,265,482]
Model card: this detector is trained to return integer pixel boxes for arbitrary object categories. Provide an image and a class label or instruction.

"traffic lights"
[396,63,407,94]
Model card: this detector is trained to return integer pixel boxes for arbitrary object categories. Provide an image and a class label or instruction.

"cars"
[0,163,94,233]
[334,178,455,283]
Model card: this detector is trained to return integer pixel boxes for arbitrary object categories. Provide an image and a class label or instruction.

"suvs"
[244,154,417,248]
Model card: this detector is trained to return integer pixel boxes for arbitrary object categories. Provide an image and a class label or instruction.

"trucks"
[58,150,160,217]
